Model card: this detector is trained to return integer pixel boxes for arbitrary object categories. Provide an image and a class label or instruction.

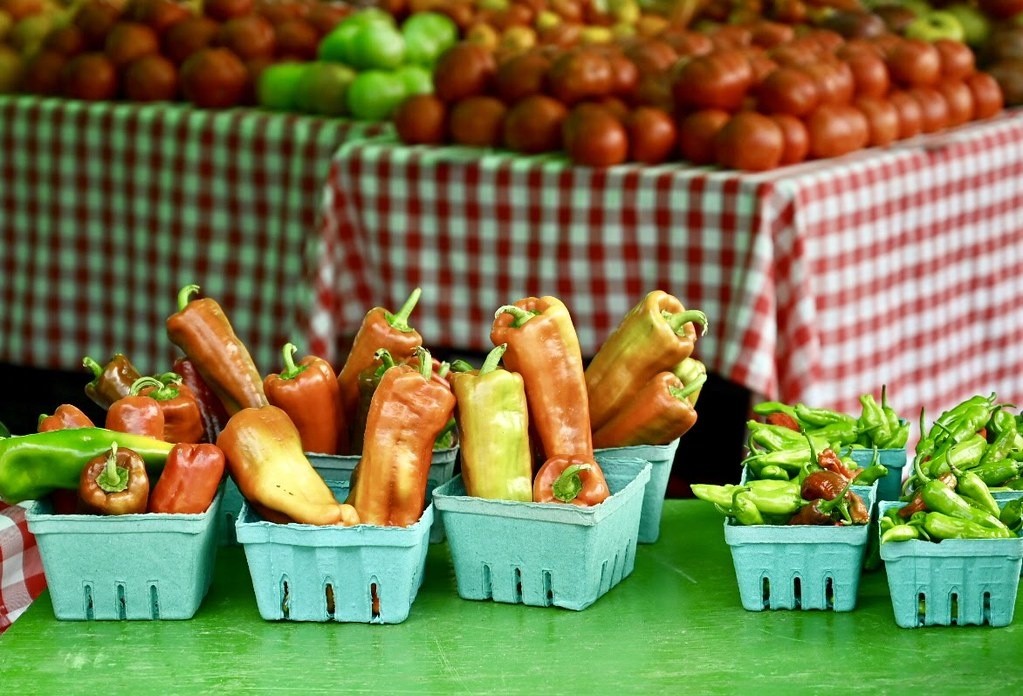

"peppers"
[0,284,1023,616]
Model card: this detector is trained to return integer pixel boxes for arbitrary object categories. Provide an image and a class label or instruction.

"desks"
[1,93,1023,487]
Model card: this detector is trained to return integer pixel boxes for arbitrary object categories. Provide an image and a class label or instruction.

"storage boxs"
[22,431,1023,628]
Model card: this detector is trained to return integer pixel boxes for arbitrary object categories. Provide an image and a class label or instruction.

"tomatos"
[0,0,1023,174]
